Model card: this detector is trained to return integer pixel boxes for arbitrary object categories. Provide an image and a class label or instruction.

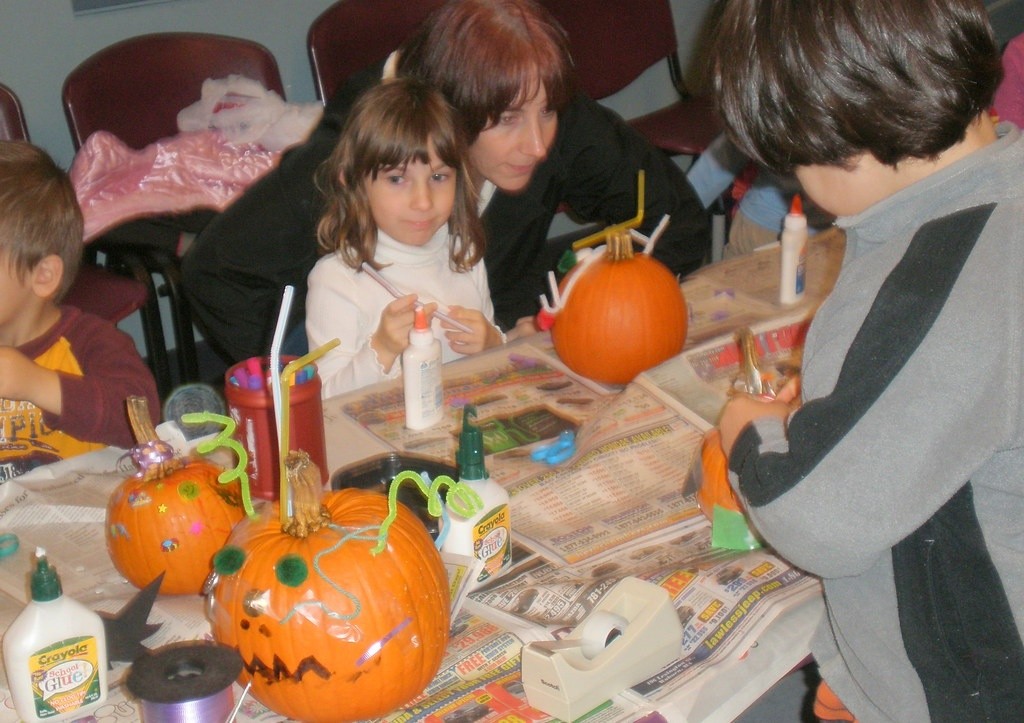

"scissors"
[531,427,575,463]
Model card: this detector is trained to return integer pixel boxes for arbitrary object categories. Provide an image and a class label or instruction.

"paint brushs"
[229,354,314,389]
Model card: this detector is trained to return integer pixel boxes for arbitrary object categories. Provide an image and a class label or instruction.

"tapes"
[578,609,627,658]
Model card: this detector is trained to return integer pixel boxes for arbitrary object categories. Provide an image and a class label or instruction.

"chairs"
[0,0,430,391]
[551,0,757,262]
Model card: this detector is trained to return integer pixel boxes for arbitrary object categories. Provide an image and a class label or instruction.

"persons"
[306,81,508,400]
[175,0,713,369]
[0,141,162,481]
[688,0,1024,723]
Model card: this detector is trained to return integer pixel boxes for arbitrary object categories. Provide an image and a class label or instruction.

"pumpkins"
[202,450,451,723]
[108,456,247,597]
[551,249,690,385]
[691,426,745,524]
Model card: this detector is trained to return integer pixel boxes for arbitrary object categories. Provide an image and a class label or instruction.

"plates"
[332,450,488,541]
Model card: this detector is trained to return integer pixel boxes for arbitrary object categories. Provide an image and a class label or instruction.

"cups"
[226,355,328,499]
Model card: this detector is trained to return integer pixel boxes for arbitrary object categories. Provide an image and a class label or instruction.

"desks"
[0,225,847,723]
